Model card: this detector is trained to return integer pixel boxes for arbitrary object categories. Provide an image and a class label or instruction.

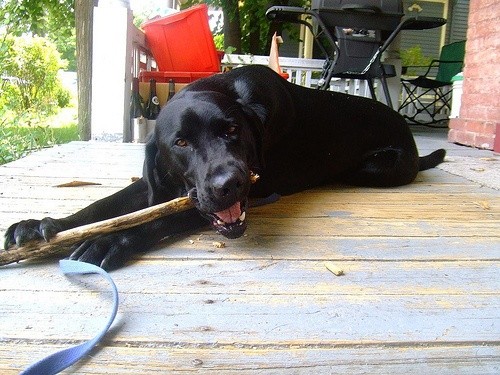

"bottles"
[166,79,175,102]
[145,79,161,140]
[130,77,145,142]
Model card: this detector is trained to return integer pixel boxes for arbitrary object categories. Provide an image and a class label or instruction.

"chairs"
[398,40,467,128]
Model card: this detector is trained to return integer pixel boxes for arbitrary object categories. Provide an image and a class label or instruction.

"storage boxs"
[139,4,289,109]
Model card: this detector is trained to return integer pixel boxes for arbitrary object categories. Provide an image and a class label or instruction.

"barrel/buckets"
[451,72,463,118]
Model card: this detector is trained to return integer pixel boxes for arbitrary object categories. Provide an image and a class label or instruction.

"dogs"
[5,65,446,277]
